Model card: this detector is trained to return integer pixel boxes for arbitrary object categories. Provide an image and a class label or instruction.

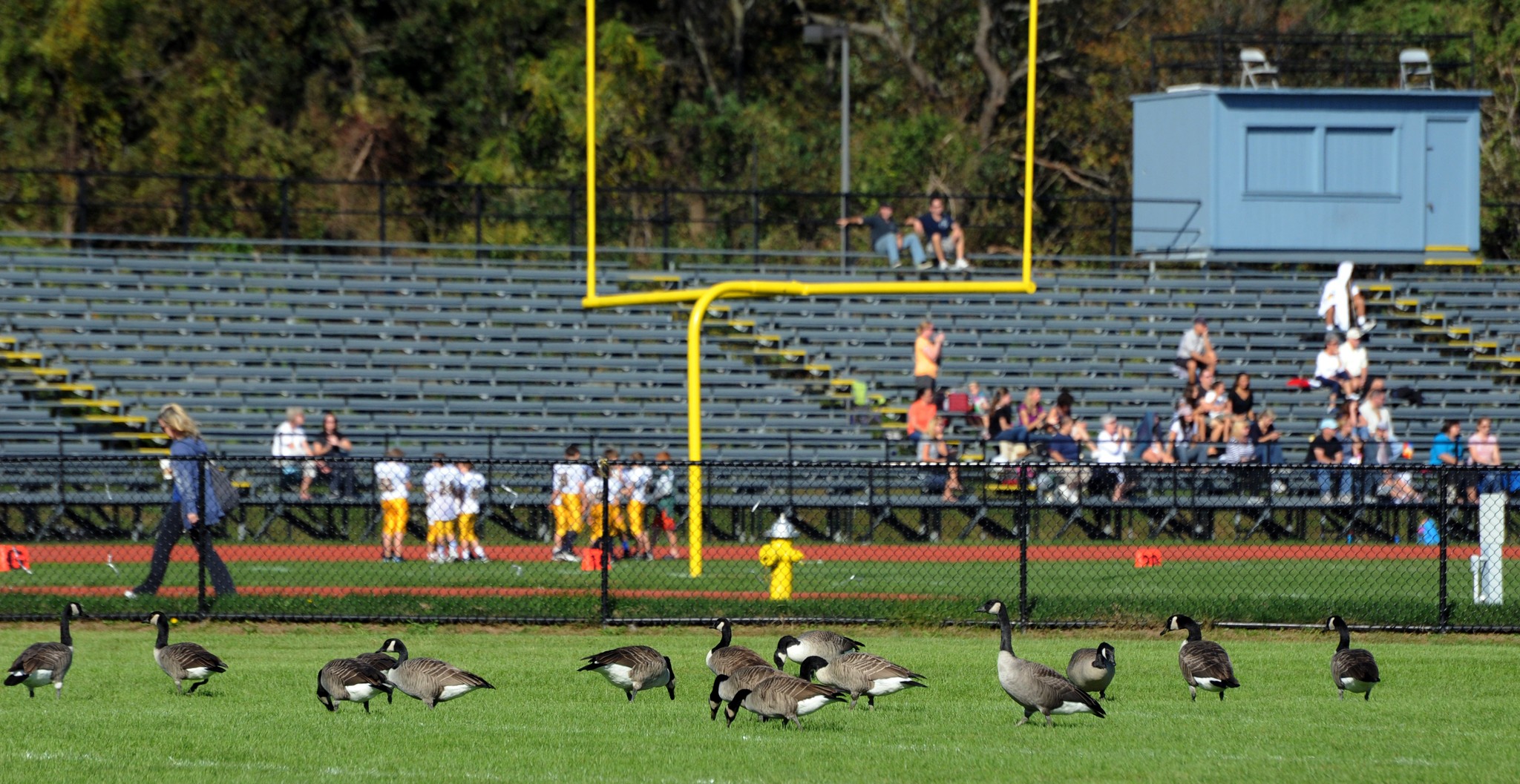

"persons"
[125,402,240,598]
[913,322,946,400]
[313,414,361,500]
[904,199,971,270]
[1176,318,1218,384]
[1319,262,1376,336]
[1337,327,1383,397]
[272,407,331,500]
[1315,333,1360,414]
[905,375,1509,501]
[370,447,685,570]
[837,203,933,270]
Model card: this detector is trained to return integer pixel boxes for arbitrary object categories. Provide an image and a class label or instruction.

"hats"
[1319,417,1340,429]
[1346,326,1365,340]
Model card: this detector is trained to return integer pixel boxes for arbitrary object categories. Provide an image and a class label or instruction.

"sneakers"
[550,550,680,563]
[383,556,405,564]
[428,552,490,565]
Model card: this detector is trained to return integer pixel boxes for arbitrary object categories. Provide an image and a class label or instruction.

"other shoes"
[123,590,137,600]
[939,257,970,271]
[891,262,902,269]
[915,260,933,270]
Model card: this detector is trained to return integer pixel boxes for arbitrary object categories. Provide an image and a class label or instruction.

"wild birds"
[797,652,929,711]
[973,600,1107,726]
[1320,615,1382,701]
[705,617,773,676]
[141,611,229,695]
[1160,614,1240,702]
[576,645,676,703]
[4,601,93,700]
[724,676,852,731]
[375,638,496,707]
[316,658,397,716]
[708,665,797,722]
[773,630,866,672]
[1066,642,1117,699]
[354,653,399,704]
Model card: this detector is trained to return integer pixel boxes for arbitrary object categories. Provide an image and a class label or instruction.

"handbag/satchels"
[207,459,241,513]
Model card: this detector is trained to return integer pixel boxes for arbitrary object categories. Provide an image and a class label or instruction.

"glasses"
[159,425,167,432]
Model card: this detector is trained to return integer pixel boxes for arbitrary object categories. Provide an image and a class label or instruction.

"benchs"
[0,232,1520,546]
